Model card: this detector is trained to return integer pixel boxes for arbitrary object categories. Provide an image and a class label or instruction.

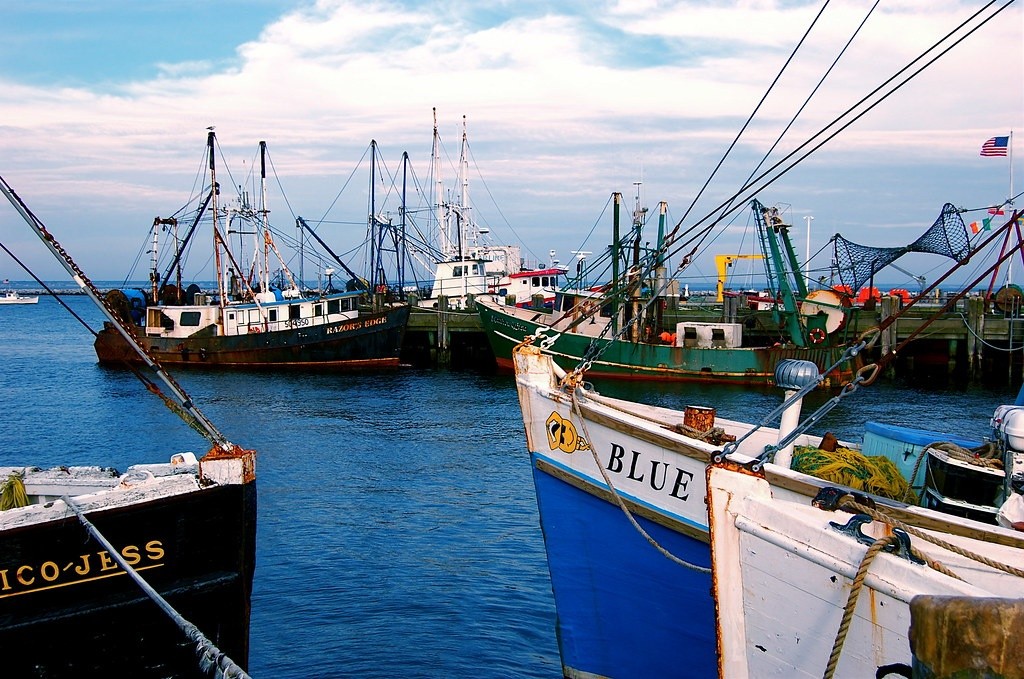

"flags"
[983,218,991,230]
[988,209,1005,215]
[970,222,978,234]
[980,136,1010,157]
[1018,214,1024,226]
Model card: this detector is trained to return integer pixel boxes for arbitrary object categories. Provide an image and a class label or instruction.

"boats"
[0,174,258,678]
[93,125,413,368]
[0,292,38,304]
[301,107,863,388]
[508,0,1023,678]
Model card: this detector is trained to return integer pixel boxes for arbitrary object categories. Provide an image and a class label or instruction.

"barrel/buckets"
[683,405,715,432]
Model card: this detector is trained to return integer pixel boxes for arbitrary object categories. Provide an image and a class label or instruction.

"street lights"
[633,182,642,210]
[803,215,814,291]
